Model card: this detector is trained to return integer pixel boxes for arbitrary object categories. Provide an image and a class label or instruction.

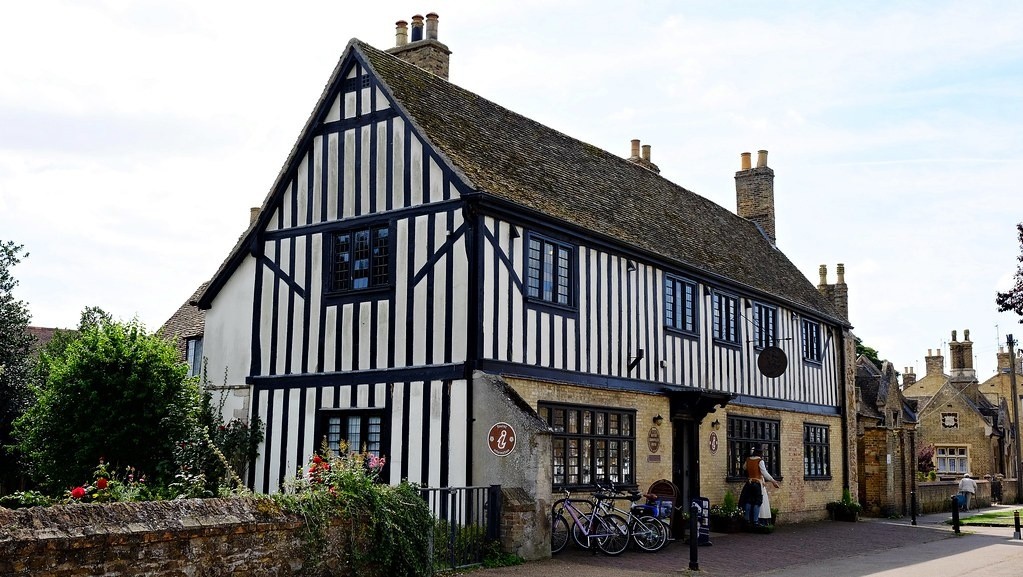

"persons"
[983,474,1003,506]
[738,448,780,528]
[959,473,978,513]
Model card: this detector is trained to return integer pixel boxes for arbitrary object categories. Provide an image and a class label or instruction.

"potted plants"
[829,488,863,523]
[710,490,779,534]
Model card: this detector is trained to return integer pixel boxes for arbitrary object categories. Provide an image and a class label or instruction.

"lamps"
[712,419,721,430]
[653,414,663,427]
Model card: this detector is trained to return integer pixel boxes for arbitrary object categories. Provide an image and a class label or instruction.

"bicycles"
[547,478,667,556]
